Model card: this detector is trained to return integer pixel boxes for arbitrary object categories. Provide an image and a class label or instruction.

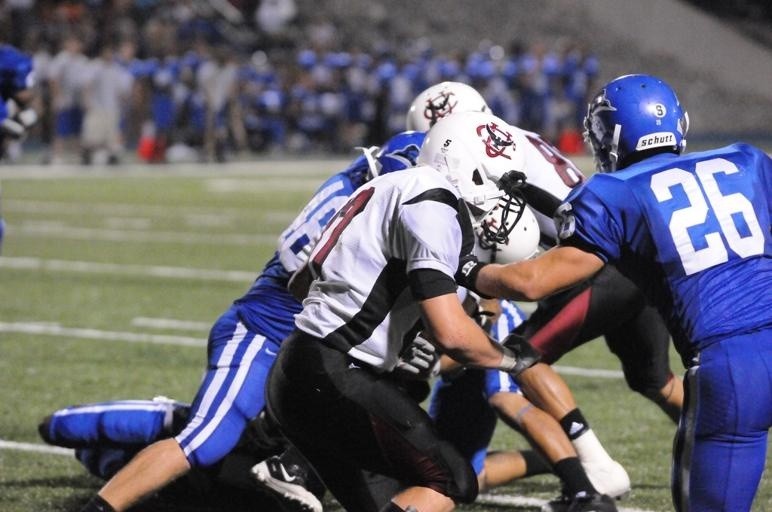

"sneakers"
[245,454,326,512]
[562,429,631,510]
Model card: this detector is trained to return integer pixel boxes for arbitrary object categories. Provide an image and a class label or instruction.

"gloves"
[502,333,540,379]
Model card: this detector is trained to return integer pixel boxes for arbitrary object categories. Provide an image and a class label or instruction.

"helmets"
[582,75,690,173]
[408,82,540,265]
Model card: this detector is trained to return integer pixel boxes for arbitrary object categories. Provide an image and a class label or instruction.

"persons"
[0,0,605,166]
[0,43,43,251]
[37,82,685,511]
[454,73,772,511]
